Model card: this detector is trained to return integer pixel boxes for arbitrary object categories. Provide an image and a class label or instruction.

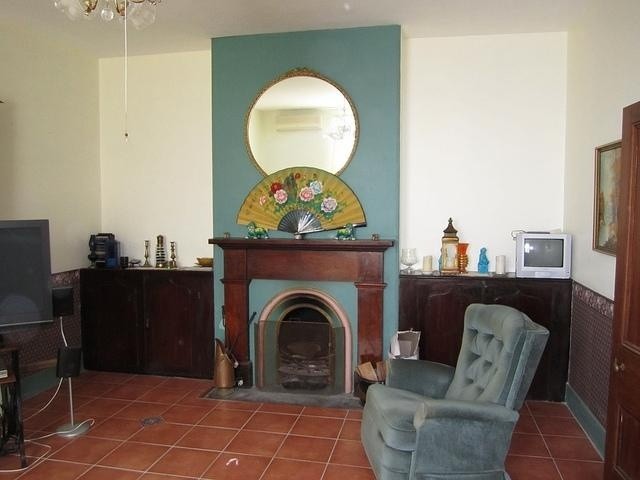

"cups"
[107,257,129,269]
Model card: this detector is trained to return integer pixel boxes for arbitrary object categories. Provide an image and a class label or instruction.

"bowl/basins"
[197,258,213,267]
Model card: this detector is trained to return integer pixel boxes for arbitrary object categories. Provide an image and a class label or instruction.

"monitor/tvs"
[516,234,571,279]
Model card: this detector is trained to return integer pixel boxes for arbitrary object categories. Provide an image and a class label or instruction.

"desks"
[0,342,28,468]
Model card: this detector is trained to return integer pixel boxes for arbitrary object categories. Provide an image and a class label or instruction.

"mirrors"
[244,68,360,179]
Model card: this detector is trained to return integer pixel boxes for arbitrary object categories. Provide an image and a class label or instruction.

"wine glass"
[400,248,417,276]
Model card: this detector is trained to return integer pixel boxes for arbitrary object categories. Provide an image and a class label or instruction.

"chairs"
[361,302,550,480]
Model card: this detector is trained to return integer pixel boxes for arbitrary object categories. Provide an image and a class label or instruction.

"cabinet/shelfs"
[79,268,214,380]
[399,275,572,402]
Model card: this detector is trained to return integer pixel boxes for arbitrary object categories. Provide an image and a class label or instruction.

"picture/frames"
[591,141,622,258]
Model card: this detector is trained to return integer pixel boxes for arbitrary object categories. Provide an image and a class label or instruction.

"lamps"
[53,1,162,141]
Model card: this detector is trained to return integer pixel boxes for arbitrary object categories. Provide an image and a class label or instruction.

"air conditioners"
[276,111,324,131]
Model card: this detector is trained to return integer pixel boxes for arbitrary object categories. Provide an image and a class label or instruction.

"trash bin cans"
[388,329,422,360]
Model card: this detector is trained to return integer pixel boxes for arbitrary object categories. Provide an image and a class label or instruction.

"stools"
[0,369,17,457]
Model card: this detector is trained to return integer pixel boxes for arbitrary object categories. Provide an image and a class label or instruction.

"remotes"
[527,232,550,234]
